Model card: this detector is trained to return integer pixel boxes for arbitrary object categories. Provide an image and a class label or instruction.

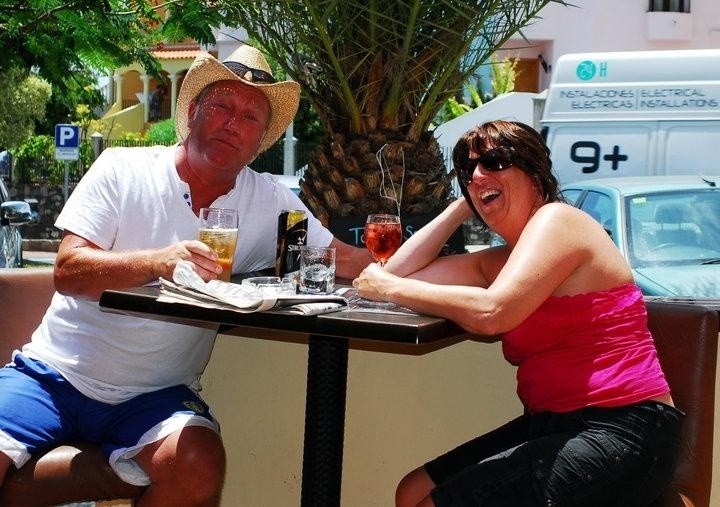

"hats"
[175,44,302,167]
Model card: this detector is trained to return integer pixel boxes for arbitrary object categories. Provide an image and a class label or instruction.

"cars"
[551,175,720,301]
[1,179,40,269]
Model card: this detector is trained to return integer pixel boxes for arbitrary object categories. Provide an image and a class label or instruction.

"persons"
[1,44,378,506]
[352,118,692,505]
[149,84,165,122]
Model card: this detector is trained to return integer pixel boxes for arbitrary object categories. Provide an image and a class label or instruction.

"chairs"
[634,299,718,505]
[0,266,149,504]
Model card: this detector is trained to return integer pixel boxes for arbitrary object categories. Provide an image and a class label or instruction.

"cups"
[364,213,403,268]
[198,207,239,285]
[300,246,336,293]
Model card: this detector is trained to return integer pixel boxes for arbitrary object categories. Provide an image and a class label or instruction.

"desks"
[96,262,472,507]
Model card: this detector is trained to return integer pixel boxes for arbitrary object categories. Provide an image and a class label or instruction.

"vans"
[541,49,720,173]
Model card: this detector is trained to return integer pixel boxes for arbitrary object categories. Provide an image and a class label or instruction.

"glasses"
[459,149,516,188]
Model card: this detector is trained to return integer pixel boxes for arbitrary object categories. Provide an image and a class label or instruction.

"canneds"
[275,210,308,280]
